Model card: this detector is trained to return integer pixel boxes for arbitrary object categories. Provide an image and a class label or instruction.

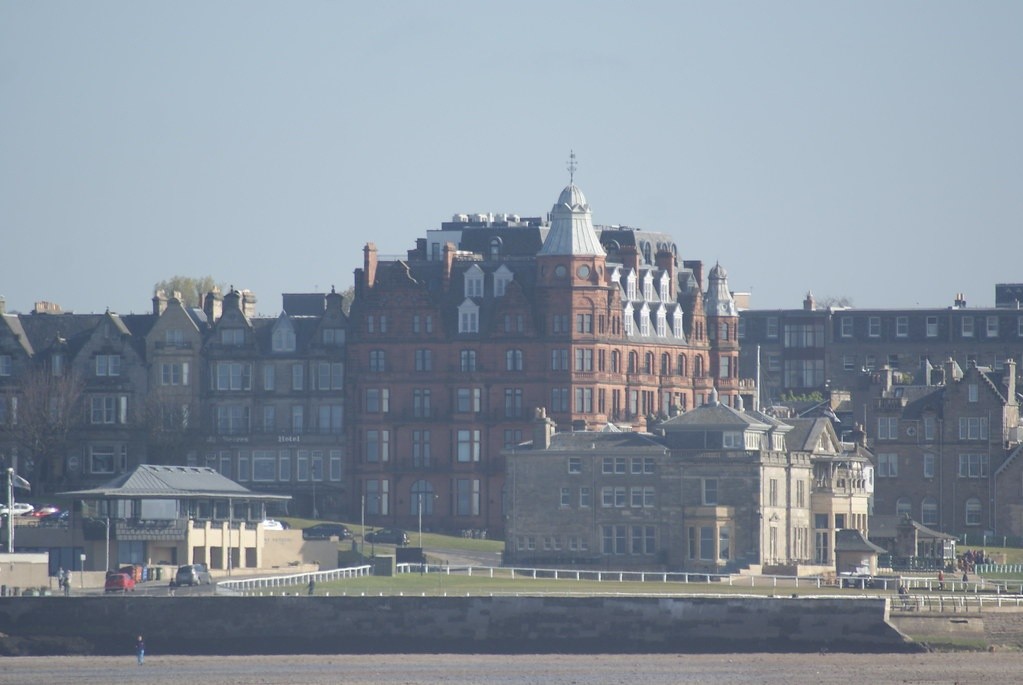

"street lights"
[78,551,87,590]
[87,513,111,572]
[950,539,955,565]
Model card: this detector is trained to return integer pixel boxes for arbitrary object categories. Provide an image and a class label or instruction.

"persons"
[308,575,315,595]
[168,578,176,597]
[135,634,147,667]
[56,564,65,591]
[899,583,909,612]
[954,548,994,573]
[962,571,969,593]
[61,569,73,597]
[936,569,944,591]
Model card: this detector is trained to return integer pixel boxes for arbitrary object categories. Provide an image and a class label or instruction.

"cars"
[301,522,353,541]
[363,529,410,546]
[104,574,135,593]
[41,509,71,523]
[175,565,212,586]
[26,506,61,519]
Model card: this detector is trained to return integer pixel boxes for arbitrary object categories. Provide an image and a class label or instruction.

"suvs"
[0,503,34,521]
